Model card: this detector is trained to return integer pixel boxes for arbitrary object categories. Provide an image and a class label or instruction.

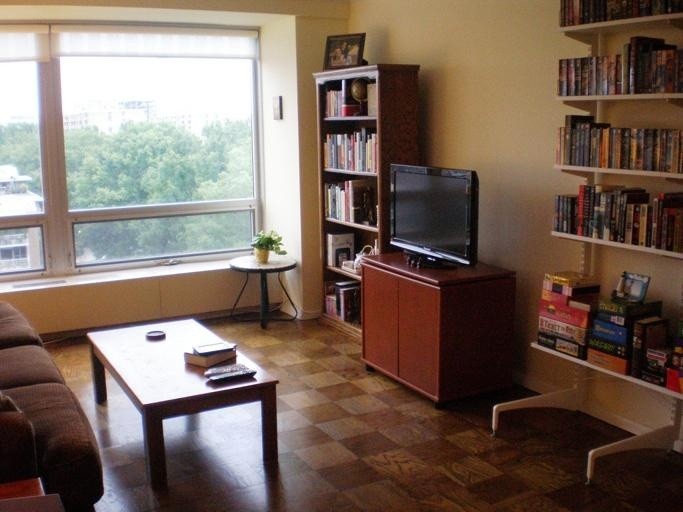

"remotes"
[204,363,249,378]
[209,368,257,384]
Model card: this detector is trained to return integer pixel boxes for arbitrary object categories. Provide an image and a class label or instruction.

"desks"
[229,256,297,329]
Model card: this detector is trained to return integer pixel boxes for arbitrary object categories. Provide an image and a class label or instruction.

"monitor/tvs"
[389,163,479,270]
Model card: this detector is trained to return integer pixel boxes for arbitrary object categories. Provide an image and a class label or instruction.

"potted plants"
[250,229,287,263]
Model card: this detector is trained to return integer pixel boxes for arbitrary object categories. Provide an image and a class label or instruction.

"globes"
[350,77,372,116]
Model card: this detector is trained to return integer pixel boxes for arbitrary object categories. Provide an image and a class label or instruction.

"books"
[630,314,672,378]
[552,0,683,256]
[321,87,377,323]
[184,345,236,368]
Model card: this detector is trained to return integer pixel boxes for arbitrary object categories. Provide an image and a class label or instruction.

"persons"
[328,41,359,65]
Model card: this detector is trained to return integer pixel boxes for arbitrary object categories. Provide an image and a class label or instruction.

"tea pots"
[354,252,370,275]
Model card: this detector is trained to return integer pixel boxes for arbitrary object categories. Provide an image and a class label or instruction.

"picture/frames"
[321,31,366,71]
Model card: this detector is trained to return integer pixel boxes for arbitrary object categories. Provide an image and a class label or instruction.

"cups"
[343,260,354,270]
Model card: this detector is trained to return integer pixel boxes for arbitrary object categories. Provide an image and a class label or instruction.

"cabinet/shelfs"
[489,12,683,483]
[311,64,421,337]
[358,254,517,406]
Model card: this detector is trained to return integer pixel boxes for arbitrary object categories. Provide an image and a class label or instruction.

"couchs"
[0,299,103,510]
